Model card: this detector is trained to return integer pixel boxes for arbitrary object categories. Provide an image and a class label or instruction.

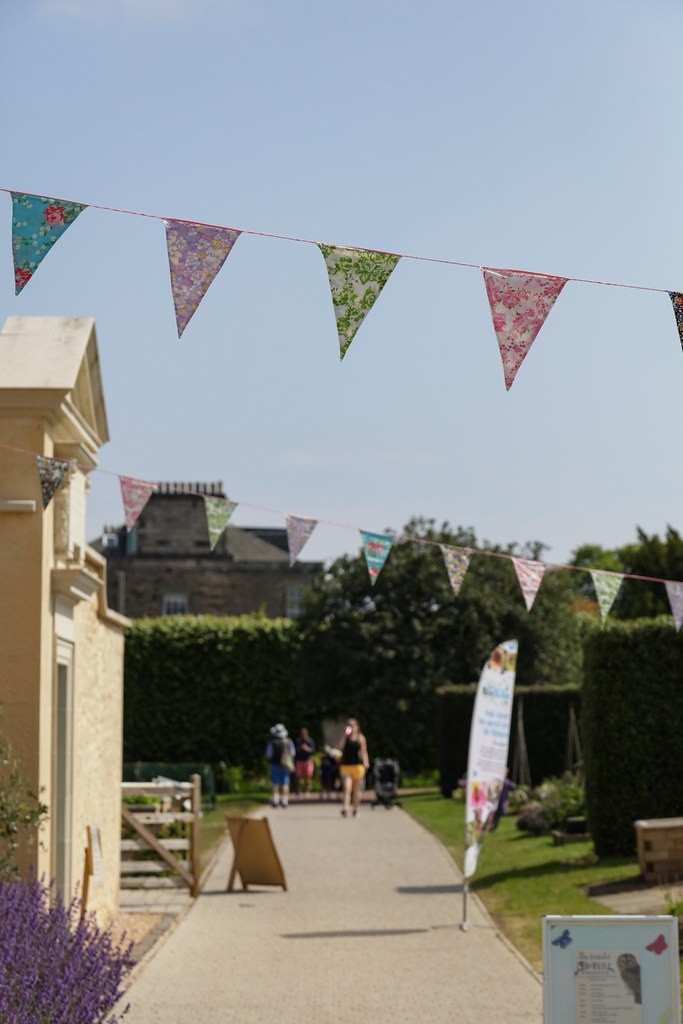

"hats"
[271,724,288,737]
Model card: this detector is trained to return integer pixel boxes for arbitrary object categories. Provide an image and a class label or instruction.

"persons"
[295,728,315,798]
[319,750,333,801]
[335,718,369,819]
[265,724,296,808]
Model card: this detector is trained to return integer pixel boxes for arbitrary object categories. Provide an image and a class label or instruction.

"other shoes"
[296,791,301,800]
[353,810,359,816]
[272,800,288,807]
[303,790,311,798]
[341,810,347,817]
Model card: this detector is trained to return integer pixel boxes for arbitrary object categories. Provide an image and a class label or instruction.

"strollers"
[372,757,400,804]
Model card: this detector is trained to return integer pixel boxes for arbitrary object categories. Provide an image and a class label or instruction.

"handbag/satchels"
[280,749,295,773]
